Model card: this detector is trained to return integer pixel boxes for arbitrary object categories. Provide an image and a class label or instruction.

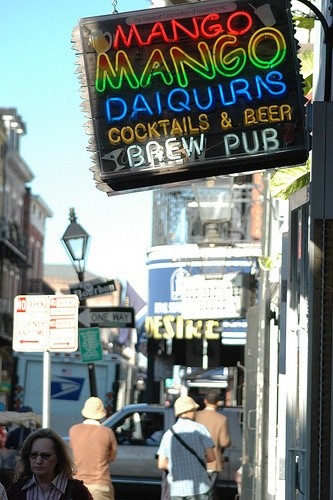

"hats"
[174,396,200,417]
[81,396,107,420]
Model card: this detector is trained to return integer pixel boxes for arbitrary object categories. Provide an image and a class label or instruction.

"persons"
[69,396,118,500]
[0,406,94,500]
[122,380,231,500]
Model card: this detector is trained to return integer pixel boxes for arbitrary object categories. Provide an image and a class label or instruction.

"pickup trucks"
[59,402,244,500]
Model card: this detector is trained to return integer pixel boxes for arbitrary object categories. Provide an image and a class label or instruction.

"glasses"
[28,452,57,460]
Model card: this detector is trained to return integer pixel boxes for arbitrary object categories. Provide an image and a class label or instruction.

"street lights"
[62,207,102,397]
